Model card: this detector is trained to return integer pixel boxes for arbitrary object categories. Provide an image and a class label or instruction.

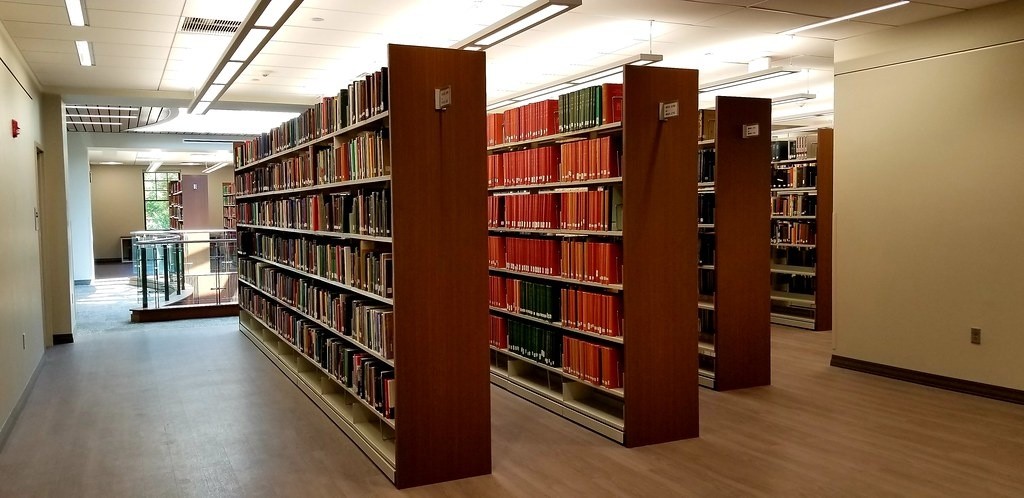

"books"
[168,182,183,230]
[234,65,396,418]
[697,110,718,391]
[223,183,237,253]
[770,139,817,312]
[486,84,624,387]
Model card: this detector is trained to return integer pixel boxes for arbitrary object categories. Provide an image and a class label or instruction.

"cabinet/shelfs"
[169,43,834,490]
